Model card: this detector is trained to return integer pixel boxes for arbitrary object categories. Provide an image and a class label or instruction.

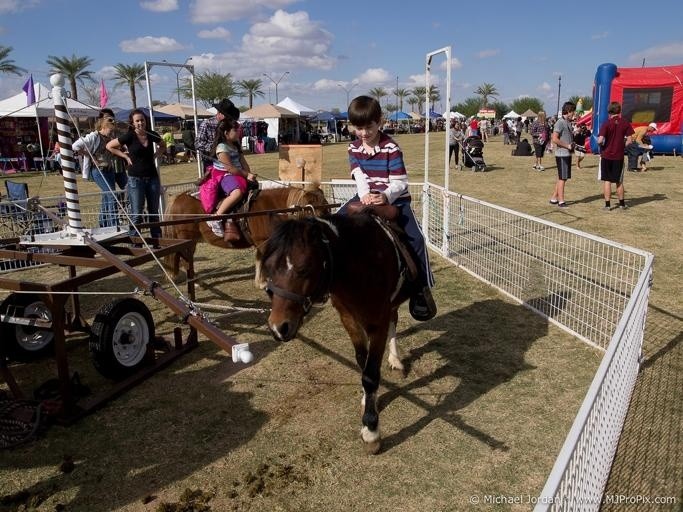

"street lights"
[337,81,358,114]
[159,57,192,103]
[262,71,290,107]
[555,73,563,117]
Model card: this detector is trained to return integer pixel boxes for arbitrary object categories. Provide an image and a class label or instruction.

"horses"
[163,174,330,289]
[259,195,417,455]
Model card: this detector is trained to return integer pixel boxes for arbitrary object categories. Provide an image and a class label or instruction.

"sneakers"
[617,204,628,210]
[549,200,569,208]
[449,164,458,170]
[576,166,581,170]
[482,139,522,145]
[532,163,544,171]
[627,167,648,172]
[599,204,611,212]
[205,219,224,238]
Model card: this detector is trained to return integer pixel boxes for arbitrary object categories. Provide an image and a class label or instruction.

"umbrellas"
[314,108,441,132]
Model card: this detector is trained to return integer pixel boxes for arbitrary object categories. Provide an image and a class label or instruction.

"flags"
[97,80,109,107]
[20,74,36,106]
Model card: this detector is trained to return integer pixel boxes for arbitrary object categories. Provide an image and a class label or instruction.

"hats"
[211,97,240,120]
[648,122,659,131]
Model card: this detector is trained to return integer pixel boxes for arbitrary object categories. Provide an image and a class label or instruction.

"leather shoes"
[407,286,437,321]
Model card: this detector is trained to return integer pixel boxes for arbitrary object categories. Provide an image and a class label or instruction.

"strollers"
[457,136,487,173]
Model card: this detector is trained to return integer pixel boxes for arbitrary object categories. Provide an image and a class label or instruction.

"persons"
[161,128,174,166]
[193,97,241,174]
[202,116,256,240]
[96,109,128,213]
[547,100,577,210]
[442,109,658,173]
[104,108,166,250]
[332,93,437,324]
[180,124,196,164]
[596,101,636,212]
[69,115,117,229]
